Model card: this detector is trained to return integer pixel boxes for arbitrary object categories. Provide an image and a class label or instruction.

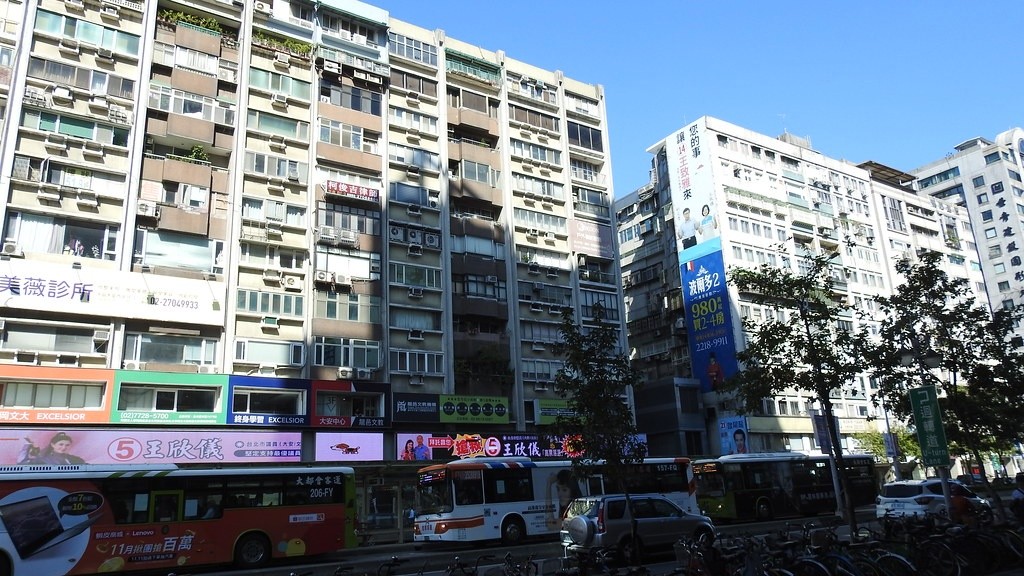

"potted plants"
[251,30,310,61]
[157,9,237,41]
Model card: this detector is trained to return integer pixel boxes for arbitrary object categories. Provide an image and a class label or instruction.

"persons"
[402,436,430,460]
[708,353,724,384]
[678,204,717,250]
[30,432,85,464]
[409,507,415,529]
[203,498,216,518]
[728,429,749,454]
[220,494,249,508]
[546,469,582,531]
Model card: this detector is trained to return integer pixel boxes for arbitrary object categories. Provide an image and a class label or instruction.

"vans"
[957,473,984,487]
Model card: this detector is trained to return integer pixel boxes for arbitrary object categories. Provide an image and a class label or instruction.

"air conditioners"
[93,330,110,342]
[136,200,157,217]
[313,270,352,287]
[123,360,141,370]
[284,275,301,290]
[325,61,380,83]
[1,243,23,256]
[254,1,270,14]
[320,95,331,104]
[275,54,288,64]
[336,366,371,379]
[319,226,355,244]
[352,33,366,46]
[813,176,870,302]
[388,74,555,291]
[197,364,214,373]
[644,329,661,362]
[97,47,112,59]
[288,170,299,181]
[274,94,286,104]
[339,29,351,41]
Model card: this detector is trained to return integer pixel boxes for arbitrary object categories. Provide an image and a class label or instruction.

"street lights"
[897,329,956,519]
[800,250,854,515]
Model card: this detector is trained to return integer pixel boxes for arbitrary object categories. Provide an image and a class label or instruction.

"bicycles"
[667,508,1024,576]
[560,540,650,576]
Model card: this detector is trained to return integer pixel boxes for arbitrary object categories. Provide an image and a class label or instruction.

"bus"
[692,453,879,522]
[413,457,699,548]
[0,463,360,576]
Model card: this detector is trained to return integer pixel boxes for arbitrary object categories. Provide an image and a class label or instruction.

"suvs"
[872,478,994,527]
[559,494,719,565]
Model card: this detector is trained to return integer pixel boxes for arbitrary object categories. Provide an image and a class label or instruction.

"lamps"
[202,272,216,280]
[71,260,83,268]
[142,262,151,271]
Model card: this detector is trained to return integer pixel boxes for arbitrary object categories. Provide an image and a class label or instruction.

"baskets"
[483,566,505,576]
[764,533,786,556]
[540,559,563,575]
[673,542,691,566]
